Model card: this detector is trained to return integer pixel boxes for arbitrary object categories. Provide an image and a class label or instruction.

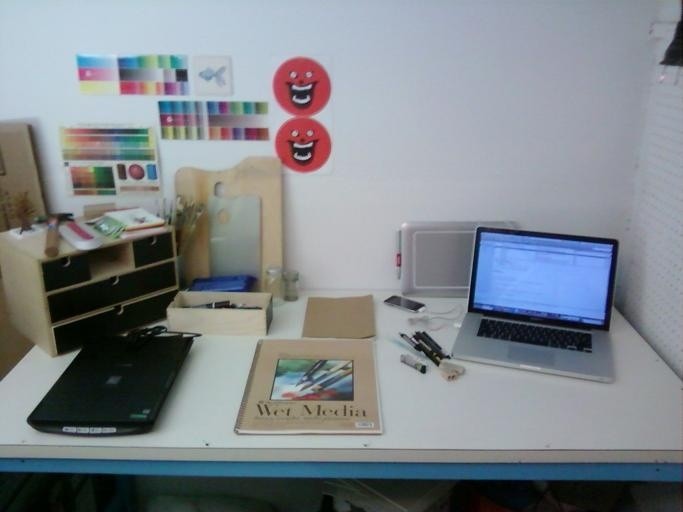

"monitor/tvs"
[301,294,377,338]
[234,338,383,438]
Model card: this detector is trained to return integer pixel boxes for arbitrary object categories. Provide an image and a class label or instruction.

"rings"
[187,300,231,309]
[397,330,447,373]
[295,361,352,390]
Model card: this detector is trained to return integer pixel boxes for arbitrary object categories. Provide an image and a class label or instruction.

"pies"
[156,195,205,257]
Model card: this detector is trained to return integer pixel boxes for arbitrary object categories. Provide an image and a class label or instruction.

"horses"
[385,293,427,315]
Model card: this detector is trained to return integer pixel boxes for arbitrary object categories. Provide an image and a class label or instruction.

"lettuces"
[34,212,74,257]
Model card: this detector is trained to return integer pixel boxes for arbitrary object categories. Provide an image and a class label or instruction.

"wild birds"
[448,225,621,384]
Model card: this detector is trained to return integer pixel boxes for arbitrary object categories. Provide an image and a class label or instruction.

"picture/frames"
[0,289,683,511]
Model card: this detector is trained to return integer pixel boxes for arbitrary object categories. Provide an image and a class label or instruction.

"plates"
[0,216,179,357]
